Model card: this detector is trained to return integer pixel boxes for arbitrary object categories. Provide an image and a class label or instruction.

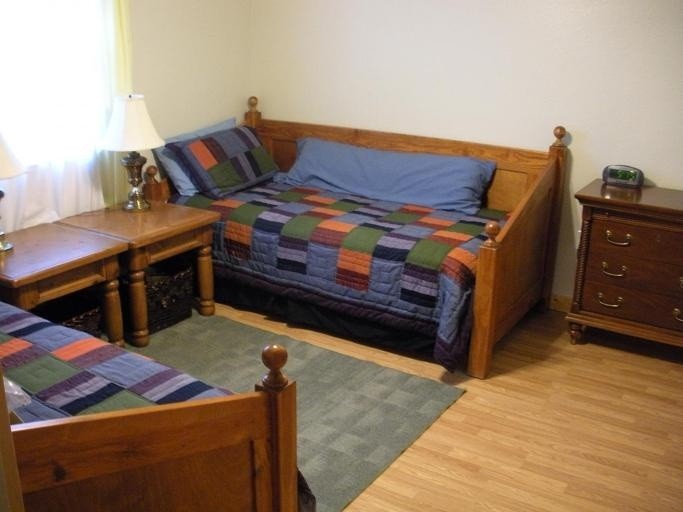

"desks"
[0,224,129,347]
[53,199,221,347]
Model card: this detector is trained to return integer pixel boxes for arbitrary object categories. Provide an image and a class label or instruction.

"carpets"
[101,306,466,512]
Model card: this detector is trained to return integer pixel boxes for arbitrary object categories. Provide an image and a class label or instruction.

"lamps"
[0,132,27,255]
[97,94,166,213]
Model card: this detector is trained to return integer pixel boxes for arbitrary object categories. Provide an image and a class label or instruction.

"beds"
[0,300,324,512]
[144,97,568,380]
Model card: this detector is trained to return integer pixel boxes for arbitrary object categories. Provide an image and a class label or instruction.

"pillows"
[165,125,280,201]
[274,137,497,215]
[151,117,236,197]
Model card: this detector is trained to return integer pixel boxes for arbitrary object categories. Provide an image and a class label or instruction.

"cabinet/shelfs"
[564,178,683,348]
